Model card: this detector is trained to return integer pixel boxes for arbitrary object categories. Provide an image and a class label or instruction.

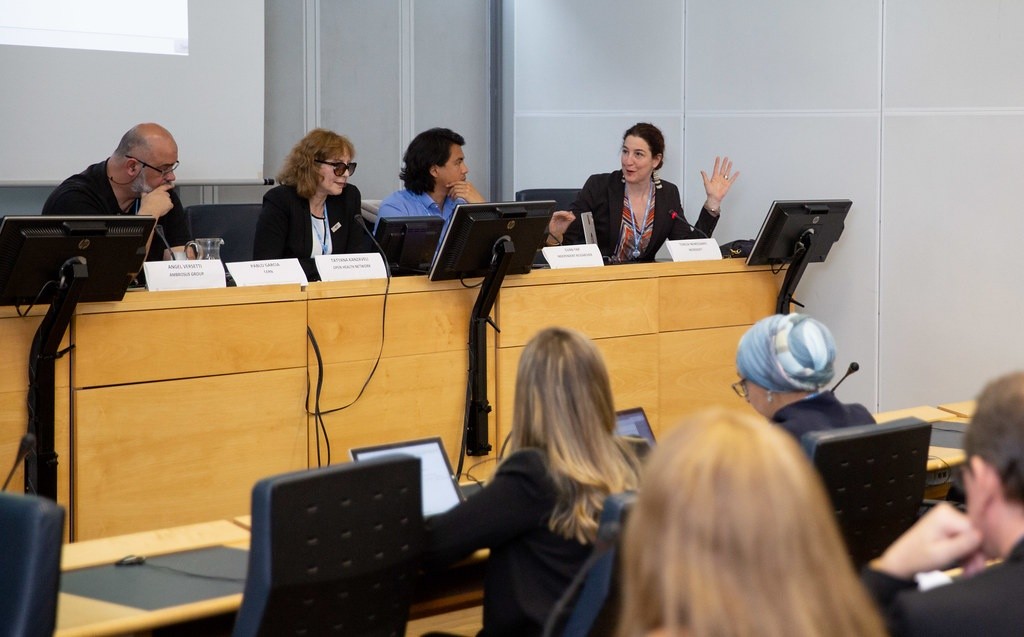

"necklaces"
[626,179,653,258]
[309,204,328,253]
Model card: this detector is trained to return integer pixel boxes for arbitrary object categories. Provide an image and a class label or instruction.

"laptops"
[348,436,465,519]
[612,406,657,447]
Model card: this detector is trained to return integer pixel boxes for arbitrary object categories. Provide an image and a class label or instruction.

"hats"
[736,313,836,392]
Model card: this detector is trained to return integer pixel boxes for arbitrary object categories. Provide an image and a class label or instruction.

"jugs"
[184,238,224,260]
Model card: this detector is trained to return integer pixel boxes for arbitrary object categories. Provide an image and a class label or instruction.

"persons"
[617,405,886,637]
[42,123,196,285]
[860,372,1024,637]
[373,127,486,265]
[422,326,639,637]
[545,122,739,266]
[252,128,362,282]
[737,313,876,442]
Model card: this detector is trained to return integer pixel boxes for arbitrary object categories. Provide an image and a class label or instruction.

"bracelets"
[547,237,563,245]
[704,201,720,213]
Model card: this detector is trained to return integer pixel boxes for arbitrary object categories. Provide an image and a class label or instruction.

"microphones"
[353,214,390,278]
[668,209,709,239]
[829,362,859,393]
[154,225,175,260]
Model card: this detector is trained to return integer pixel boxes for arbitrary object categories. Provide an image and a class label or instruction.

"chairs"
[233,454,423,637]
[804,415,932,572]
[562,491,634,636]
[186,203,264,263]
[516,189,581,264]
[0,493,65,637]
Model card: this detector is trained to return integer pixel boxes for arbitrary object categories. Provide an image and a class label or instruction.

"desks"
[52,397,970,637]
[1,259,796,544]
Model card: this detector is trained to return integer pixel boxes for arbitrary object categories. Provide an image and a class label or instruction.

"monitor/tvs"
[427,200,558,281]
[746,199,853,266]
[372,216,445,276]
[0,215,158,307]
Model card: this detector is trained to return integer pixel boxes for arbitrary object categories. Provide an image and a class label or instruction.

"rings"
[724,175,729,179]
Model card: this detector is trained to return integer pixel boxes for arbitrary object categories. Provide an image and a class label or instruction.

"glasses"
[732,378,746,397]
[314,159,357,176]
[125,154,179,178]
